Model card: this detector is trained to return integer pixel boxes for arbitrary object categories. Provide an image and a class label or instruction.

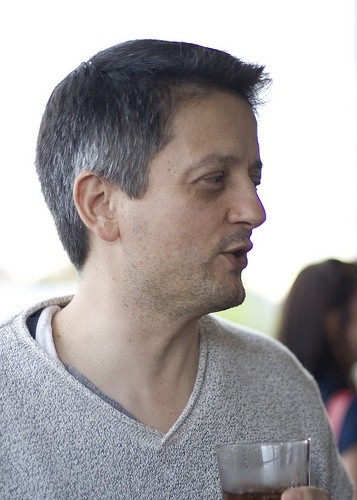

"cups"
[215,438,310,500]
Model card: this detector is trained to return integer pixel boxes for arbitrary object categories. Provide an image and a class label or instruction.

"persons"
[276,259,357,500]
[0,40,353,500]
